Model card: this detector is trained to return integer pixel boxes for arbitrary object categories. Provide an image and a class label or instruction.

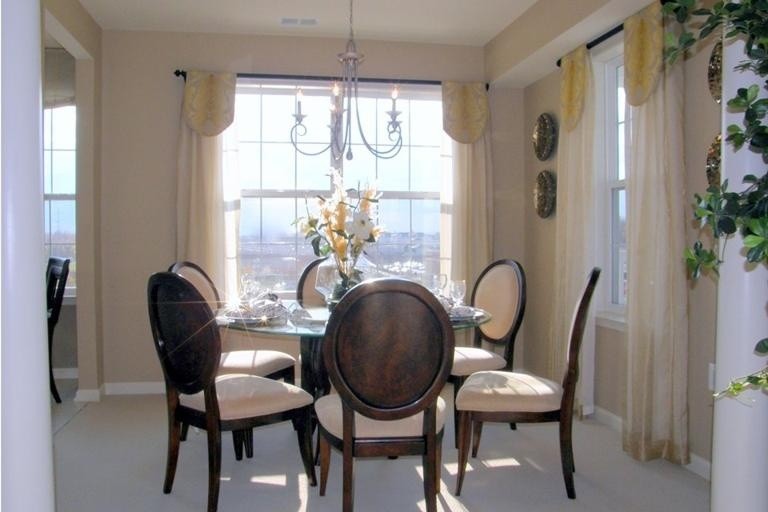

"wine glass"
[432,273,466,305]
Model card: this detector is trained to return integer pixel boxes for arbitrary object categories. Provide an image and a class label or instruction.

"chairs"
[454,266,602,503]
[443,260,527,449]
[146,271,316,512]
[314,277,455,512]
[164,260,298,460]
[297,257,362,395]
[46,257,72,404]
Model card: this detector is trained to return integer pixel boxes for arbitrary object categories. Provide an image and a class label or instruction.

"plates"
[224,310,280,324]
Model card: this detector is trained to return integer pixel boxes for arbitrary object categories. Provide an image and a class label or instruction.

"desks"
[212,298,493,464]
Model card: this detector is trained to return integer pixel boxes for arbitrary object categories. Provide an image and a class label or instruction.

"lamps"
[288,1,405,162]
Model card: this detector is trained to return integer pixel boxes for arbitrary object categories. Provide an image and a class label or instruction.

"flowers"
[291,165,386,287]
